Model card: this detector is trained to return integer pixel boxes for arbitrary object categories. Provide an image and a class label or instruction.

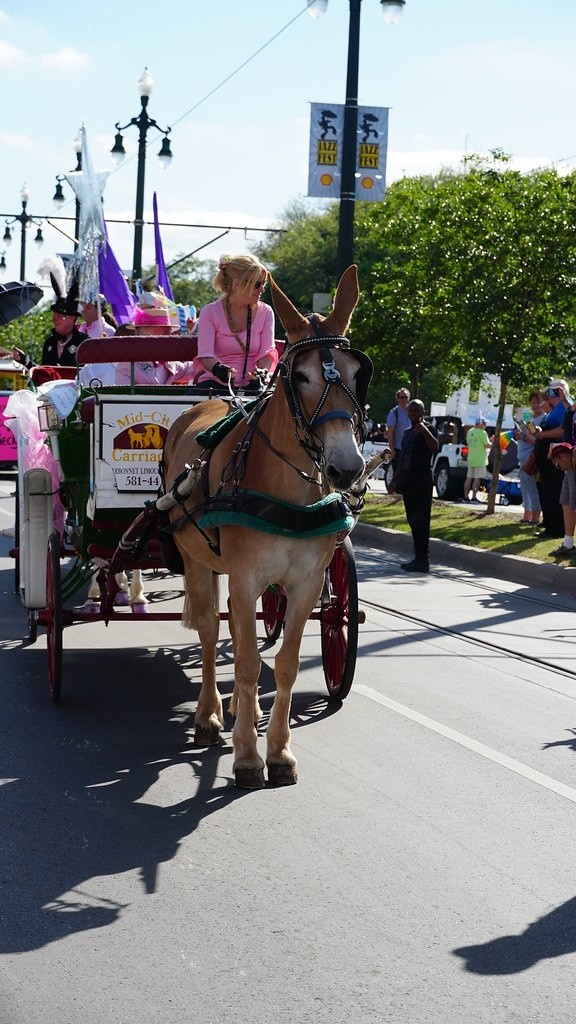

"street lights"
[308,0,404,368]
[53,127,106,286]
[107,64,177,303]
[4,181,45,284]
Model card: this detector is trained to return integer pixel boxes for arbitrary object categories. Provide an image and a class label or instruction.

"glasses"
[555,458,561,470]
[398,396,409,399]
[239,277,267,289]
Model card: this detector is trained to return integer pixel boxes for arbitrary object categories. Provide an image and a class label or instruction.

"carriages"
[8,261,370,792]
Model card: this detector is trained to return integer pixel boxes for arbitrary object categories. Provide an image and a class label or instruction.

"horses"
[159,263,365,789]
[81,568,148,613]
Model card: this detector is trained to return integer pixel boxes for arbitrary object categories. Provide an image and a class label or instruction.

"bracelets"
[523,435,527,442]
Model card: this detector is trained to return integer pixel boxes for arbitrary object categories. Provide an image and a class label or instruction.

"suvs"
[383,403,525,506]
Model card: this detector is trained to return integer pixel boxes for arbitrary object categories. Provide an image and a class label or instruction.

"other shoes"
[534,529,566,539]
[515,517,540,525]
[547,544,576,557]
[461,498,485,503]
[538,522,548,528]
[401,558,430,572]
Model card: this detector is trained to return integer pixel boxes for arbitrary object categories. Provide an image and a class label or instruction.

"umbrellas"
[0,281,43,368]
[491,430,514,451]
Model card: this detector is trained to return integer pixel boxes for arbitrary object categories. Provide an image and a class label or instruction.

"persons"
[13,297,92,368]
[548,382,576,558]
[461,416,492,504]
[530,379,574,538]
[387,388,439,572]
[76,324,135,387]
[194,254,278,390]
[550,443,576,472]
[515,390,552,527]
[79,293,116,336]
[117,309,196,385]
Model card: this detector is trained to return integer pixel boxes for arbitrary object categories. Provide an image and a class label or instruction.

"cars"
[364,419,380,442]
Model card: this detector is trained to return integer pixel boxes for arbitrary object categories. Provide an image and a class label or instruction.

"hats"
[137,292,161,309]
[50,270,81,318]
[476,416,490,424]
[76,290,106,309]
[124,308,181,333]
